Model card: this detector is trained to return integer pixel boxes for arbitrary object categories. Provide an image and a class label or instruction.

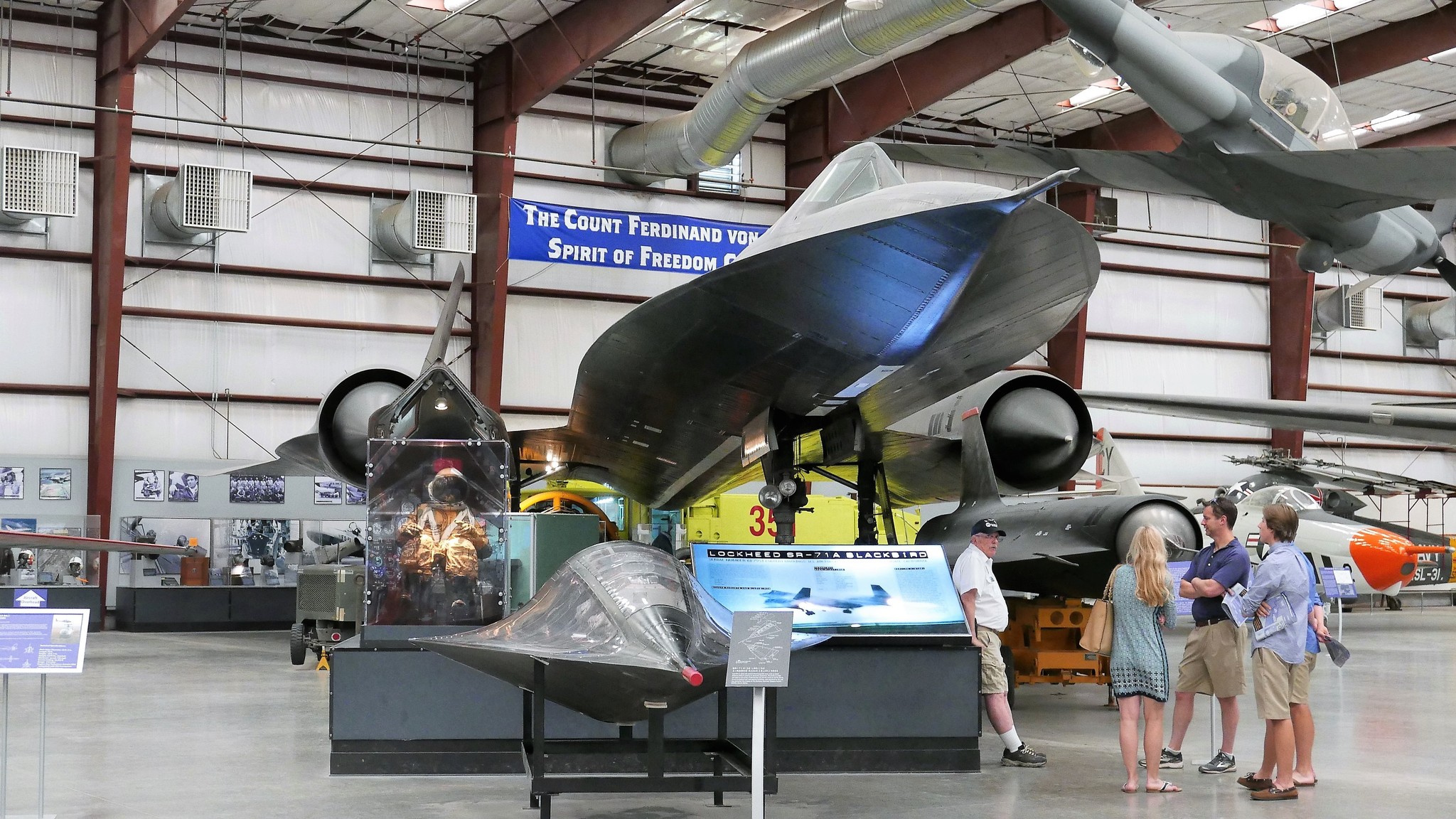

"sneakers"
[1139,746,1183,768]
[1022,741,1046,758]
[1000,745,1047,767]
[1199,749,1236,773]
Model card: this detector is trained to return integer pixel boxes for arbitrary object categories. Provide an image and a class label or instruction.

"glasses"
[1210,499,1226,513]
[975,534,1003,543]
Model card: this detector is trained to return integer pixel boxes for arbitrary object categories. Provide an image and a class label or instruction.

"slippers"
[1121,781,1139,794]
[1315,779,1318,782]
[1146,781,1182,793]
[1292,778,1315,787]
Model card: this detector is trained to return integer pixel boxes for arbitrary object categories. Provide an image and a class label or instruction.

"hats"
[971,518,1006,537]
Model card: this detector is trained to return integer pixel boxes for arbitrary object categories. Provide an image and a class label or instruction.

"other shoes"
[1237,772,1273,791]
[1250,784,1298,800]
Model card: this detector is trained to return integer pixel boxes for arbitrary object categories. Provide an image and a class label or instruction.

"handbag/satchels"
[1079,565,1123,657]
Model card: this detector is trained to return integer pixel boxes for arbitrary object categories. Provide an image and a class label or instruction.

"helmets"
[429,468,468,511]
[69,557,84,573]
[18,549,34,566]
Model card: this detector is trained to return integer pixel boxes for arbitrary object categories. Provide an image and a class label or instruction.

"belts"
[1195,616,1230,627]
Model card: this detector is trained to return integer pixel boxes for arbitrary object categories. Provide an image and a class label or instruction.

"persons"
[1109,525,1183,793]
[169,473,198,502]
[1236,503,1310,801]
[230,476,285,502]
[951,518,1048,767]
[141,476,162,499]
[395,466,489,624]
[1138,498,1250,774]
[1275,548,1331,788]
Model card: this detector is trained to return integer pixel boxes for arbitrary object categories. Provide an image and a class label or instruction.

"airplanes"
[206,0,1456,723]
